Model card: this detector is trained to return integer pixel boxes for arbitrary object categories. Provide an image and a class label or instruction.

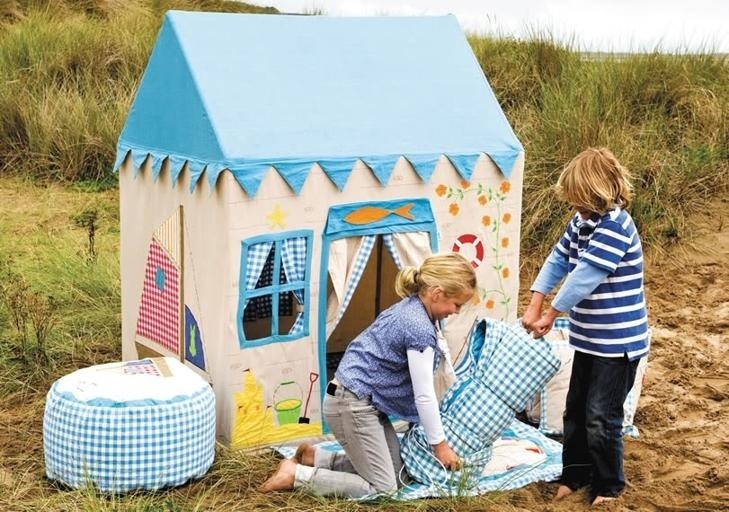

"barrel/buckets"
[271,380,304,422]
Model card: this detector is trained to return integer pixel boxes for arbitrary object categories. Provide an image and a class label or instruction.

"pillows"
[525,316,650,438]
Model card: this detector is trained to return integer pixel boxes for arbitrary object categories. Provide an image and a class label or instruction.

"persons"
[515,143,651,509]
[254,251,479,503]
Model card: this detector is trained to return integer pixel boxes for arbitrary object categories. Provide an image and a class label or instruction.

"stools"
[42,357,216,497]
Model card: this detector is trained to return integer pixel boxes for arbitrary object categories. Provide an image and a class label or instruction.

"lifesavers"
[454,236,484,269]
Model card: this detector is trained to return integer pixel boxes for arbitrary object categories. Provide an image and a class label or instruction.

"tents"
[109,8,528,464]
